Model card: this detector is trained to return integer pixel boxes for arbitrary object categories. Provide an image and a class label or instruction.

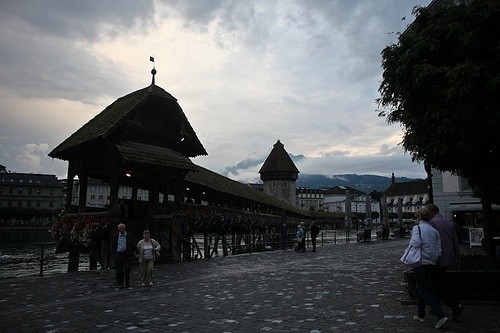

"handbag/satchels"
[399,244,423,266]
[296,237,303,243]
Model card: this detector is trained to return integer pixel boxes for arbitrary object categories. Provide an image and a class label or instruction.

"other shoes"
[311,250,316,252]
[141,283,146,287]
[434,316,448,329]
[149,282,154,287]
[412,315,425,323]
[127,286,134,290]
[429,310,444,316]
[451,302,464,323]
[113,288,120,293]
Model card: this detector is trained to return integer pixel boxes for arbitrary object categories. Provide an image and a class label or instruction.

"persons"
[427,204,464,321]
[112,224,139,290]
[297,222,307,253]
[309,221,318,252]
[364,216,373,242]
[410,208,448,329]
[134,230,161,287]
[382,217,404,239]
